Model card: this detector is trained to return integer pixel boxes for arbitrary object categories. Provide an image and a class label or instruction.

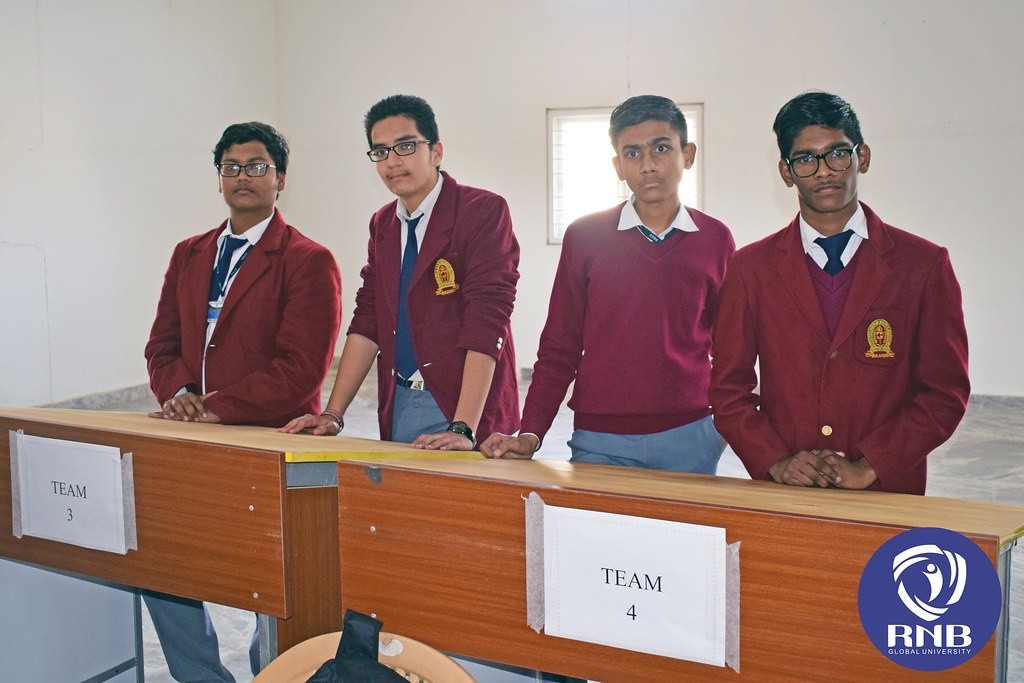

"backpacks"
[304,609,411,683]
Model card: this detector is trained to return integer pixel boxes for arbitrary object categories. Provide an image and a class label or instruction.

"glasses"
[216,163,277,177]
[366,140,430,162]
[784,144,860,178]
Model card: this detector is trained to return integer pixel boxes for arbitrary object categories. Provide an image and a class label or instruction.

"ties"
[813,230,855,276]
[394,213,426,380]
[205,235,249,329]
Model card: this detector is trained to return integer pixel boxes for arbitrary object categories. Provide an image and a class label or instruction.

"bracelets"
[320,411,345,428]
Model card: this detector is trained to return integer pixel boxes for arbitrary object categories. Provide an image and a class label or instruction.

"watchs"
[446,421,476,443]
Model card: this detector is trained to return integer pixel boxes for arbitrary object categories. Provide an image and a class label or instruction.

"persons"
[132,122,342,682]
[278,95,520,452]
[480,95,739,478]
[709,92,972,497]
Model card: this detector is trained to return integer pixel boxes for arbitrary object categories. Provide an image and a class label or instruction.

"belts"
[395,375,430,392]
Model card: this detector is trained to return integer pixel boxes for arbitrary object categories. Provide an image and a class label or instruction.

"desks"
[0,405,1024,682]
